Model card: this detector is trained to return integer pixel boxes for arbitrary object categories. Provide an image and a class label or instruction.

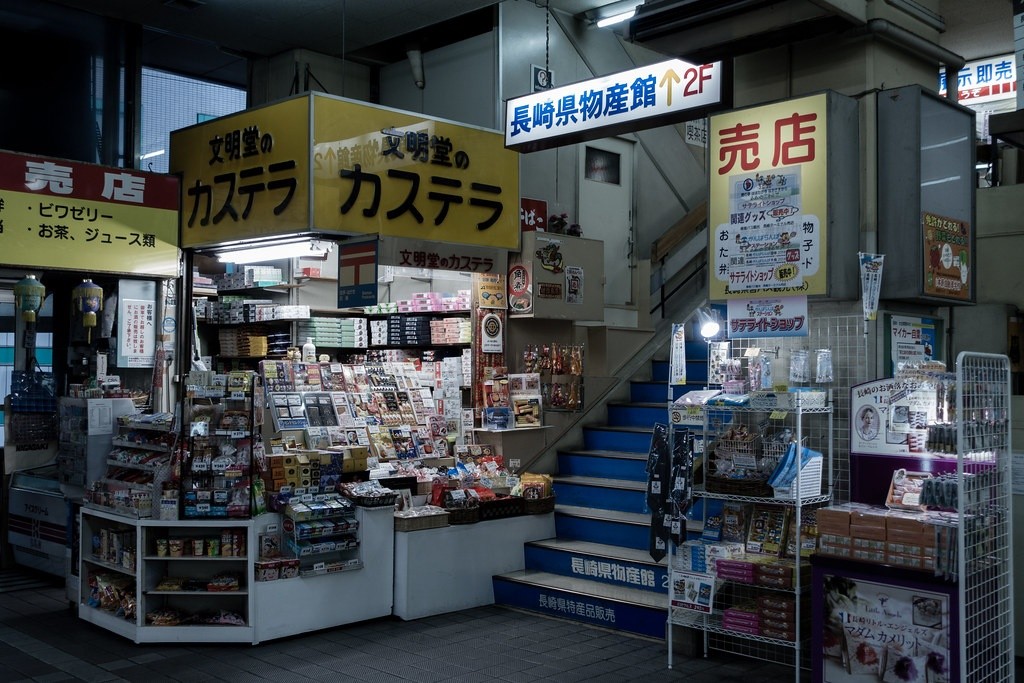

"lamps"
[407,50,424,88]
[694,306,720,343]
[574,0,645,30]
[215,240,335,265]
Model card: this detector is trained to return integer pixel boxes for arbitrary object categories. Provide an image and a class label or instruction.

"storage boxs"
[676,385,1004,642]
[90,266,585,592]
[8,370,58,451]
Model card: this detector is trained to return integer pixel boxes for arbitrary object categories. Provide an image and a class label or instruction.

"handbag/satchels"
[10,357,57,413]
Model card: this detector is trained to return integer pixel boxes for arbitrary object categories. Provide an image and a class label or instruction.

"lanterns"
[72,278,103,327]
[14,275,45,322]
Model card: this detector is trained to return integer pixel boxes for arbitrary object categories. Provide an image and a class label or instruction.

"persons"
[860,409,876,440]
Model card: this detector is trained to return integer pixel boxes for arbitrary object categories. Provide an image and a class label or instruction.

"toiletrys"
[204,265,311,324]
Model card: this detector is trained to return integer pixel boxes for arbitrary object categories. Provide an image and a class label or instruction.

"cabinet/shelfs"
[809,350,1014,683]
[664,322,834,683]
[78,307,474,645]
